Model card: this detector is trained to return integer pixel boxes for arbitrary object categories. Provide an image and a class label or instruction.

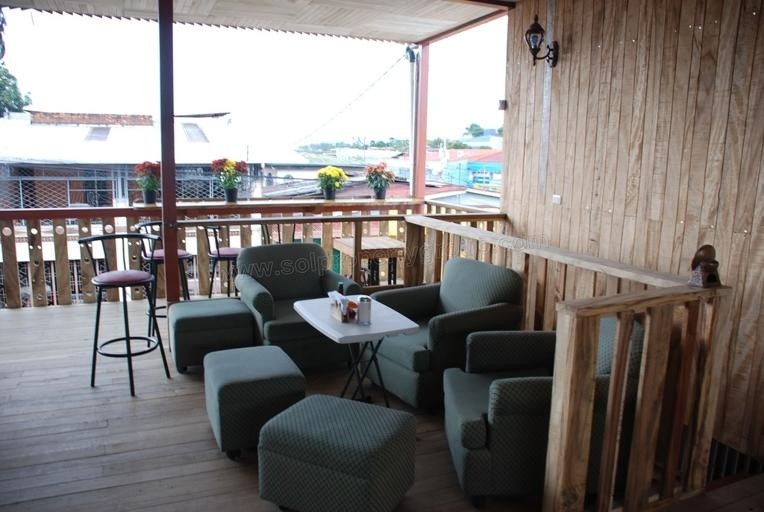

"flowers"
[365,161,396,192]
[133,161,162,192]
[316,165,351,190]
[210,158,249,190]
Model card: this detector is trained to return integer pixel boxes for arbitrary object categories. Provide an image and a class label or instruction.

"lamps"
[523,15,558,69]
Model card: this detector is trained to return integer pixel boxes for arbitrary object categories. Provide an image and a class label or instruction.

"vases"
[143,190,155,204]
[322,189,336,202]
[373,187,385,200]
[224,188,238,202]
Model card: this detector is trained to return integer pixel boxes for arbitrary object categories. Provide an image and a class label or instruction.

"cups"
[356,297,371,326]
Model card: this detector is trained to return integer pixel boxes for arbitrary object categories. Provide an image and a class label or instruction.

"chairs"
[441,316,643,509]
[199,221,243,299]
[134,221,196,348]
[365,256,522,410]
[78,233,170,397]
[234,242,359,367]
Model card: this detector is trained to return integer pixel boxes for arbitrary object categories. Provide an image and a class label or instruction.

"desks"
[293,294,420,407]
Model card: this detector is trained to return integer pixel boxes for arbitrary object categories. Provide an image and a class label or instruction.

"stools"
[202,346,305,460]
[167,298,261,373]
[256,393,417,512]
[333,236,405,283]
